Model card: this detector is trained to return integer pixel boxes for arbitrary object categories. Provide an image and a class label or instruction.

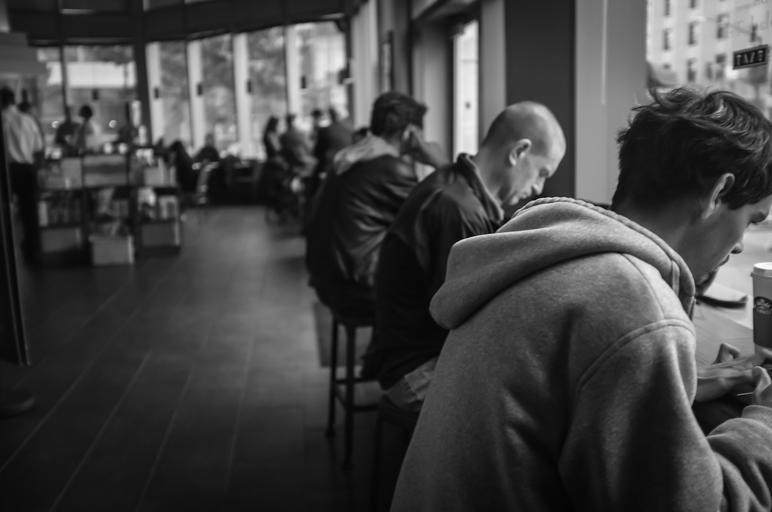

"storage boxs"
[39,155,180,266]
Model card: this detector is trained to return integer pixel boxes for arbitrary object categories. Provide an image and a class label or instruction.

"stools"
[325,314,388,472]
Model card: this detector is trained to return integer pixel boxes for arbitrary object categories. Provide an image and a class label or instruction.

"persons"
[304,90,451,382]
[367,99,569,512]
[263,105,354,223]
[1,86,224,265]
[387,82,772,511]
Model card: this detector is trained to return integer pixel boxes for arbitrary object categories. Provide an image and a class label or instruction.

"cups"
[750,262,772,315]
